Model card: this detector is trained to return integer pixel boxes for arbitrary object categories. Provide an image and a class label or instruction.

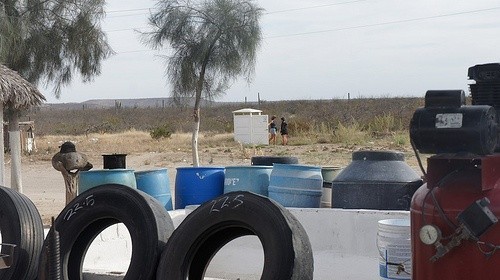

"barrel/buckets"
[78,170,137,194]
[135,170,173,211]
[321,168,342,208]
[378,219,412,280]
[268,163,323,208]
[102,154,127,169]
[332,152,422,210]
[175,167,224,209]
[225,167,273,197]
[251,156,298,167]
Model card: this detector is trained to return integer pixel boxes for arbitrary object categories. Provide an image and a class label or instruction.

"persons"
[269,116,278,142]
[280,117,289,146]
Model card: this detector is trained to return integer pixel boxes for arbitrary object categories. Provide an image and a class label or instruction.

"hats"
[281,117,286,120]
[272,115,277,119]
